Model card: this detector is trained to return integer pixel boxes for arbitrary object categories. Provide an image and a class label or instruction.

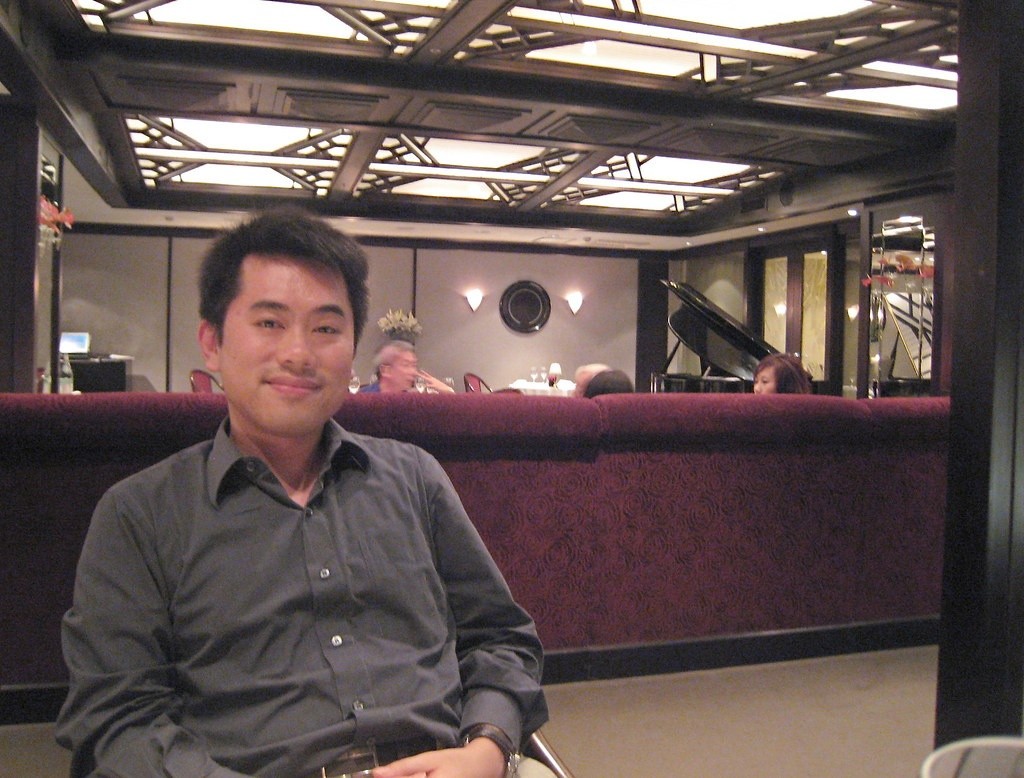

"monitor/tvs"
[59,332,89,354]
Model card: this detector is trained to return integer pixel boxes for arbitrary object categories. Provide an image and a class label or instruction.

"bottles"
[540,367,548,381]
[57,354,74,394]
[33,367,50,393]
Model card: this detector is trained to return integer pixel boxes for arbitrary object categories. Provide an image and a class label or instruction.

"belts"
[303,734,438,778]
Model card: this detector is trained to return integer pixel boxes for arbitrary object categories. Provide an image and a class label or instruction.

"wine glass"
[530,366,539,382]
[549,363,561,389]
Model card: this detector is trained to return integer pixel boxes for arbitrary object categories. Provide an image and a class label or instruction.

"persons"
[54,213,556,778]
[754,353,810,394]
[575,364,633,397]
[356,340,454,392]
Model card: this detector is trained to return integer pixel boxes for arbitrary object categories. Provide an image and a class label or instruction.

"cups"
[347,377,360,394]
[415,377,425,393]
[370,373,378,384]
[446,377,454,388]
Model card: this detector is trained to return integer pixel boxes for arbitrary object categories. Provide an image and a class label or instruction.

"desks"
[509,379,577,396]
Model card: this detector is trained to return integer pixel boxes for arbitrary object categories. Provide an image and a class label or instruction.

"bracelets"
[462,724,520,778]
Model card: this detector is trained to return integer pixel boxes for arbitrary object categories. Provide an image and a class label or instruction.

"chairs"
[190,368,224,393]
[463,372,492,395]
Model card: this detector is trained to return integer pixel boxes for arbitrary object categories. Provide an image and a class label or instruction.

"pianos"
[646,272,845,398]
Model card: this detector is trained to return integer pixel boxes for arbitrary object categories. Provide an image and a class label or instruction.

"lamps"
[568,291,583,316]
[466,288,483,310]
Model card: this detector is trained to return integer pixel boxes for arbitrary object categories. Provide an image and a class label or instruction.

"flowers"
[381,308,422,345]
